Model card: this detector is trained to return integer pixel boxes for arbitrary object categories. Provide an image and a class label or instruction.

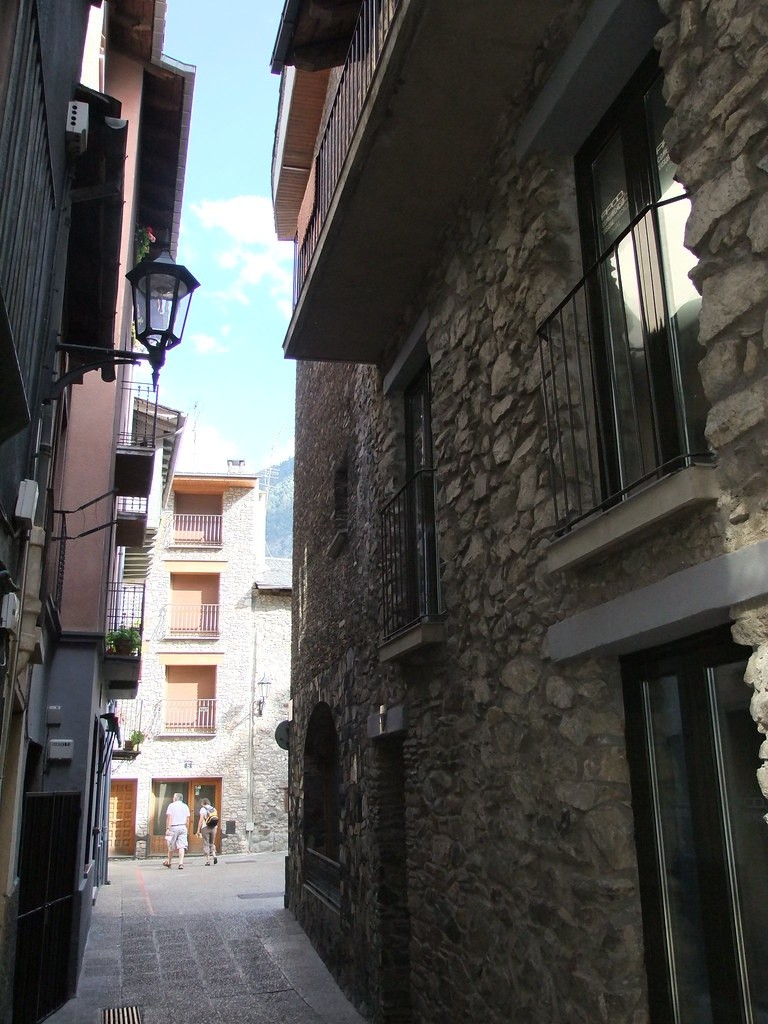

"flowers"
[136,225,163,265]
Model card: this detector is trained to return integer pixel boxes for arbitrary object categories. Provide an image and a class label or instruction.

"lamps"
[258,672,273,718]
[43,228,202,405]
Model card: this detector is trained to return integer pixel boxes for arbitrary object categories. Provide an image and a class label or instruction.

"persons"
[162,792,191,869]
[195,798,218,866]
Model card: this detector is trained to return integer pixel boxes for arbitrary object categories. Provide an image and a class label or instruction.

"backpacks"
[203,806,219,828]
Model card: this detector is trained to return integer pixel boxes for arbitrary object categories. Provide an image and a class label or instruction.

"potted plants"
[124,731,145,751]
[105,626,142,656]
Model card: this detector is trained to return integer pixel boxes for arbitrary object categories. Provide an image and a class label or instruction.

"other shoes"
[178,864,184,869]
[162,861,172,868]
[214,857,217,864]
[205,863,210,866]
